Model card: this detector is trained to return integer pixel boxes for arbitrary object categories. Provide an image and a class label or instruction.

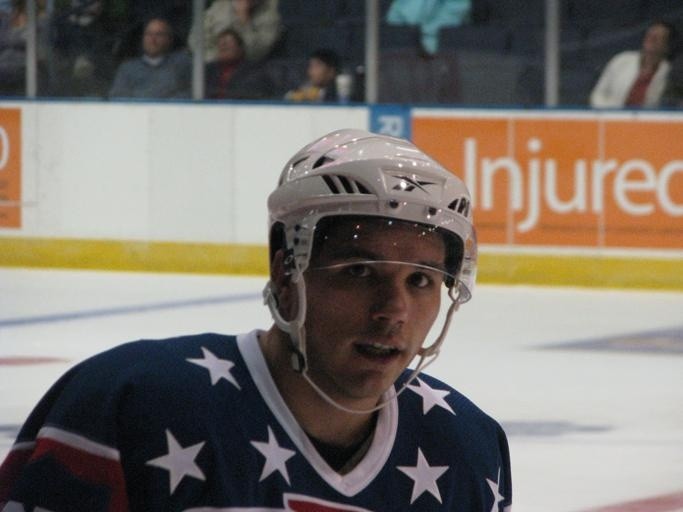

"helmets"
[262,129,478,414]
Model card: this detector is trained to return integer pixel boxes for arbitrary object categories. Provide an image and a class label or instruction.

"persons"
[587,21,673,110]
[0,123,515,511]
[284,49,355,103]
[2,1,285,101]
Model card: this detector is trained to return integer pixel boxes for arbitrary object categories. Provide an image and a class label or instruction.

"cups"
[335,73,353,106]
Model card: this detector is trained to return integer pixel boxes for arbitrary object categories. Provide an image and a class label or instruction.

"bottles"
[355,63,366,103]
